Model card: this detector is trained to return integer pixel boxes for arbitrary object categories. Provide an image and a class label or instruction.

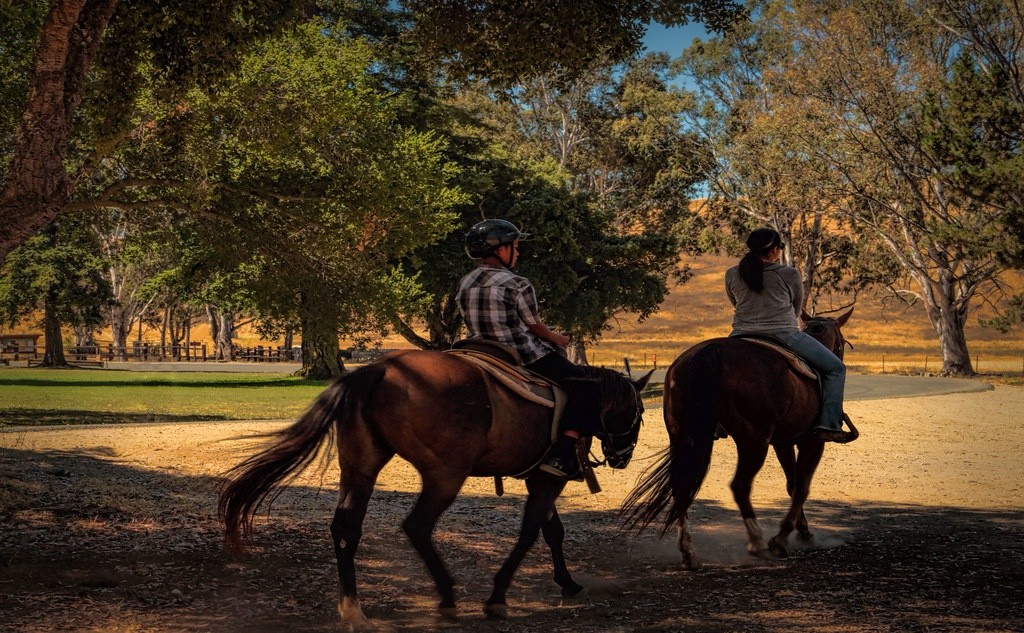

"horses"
[202,344,657,633]
[617,306,858,571]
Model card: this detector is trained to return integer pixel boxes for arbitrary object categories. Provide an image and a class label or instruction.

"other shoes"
[814,428,851,442]
[539,456,585,482]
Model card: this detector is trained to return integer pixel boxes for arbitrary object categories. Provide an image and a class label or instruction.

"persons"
[455,219,586,482]
[725,228,850,440]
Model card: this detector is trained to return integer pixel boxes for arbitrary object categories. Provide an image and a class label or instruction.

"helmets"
[465,219,531,259]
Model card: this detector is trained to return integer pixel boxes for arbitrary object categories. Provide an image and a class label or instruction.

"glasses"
[779,243,785,250]
